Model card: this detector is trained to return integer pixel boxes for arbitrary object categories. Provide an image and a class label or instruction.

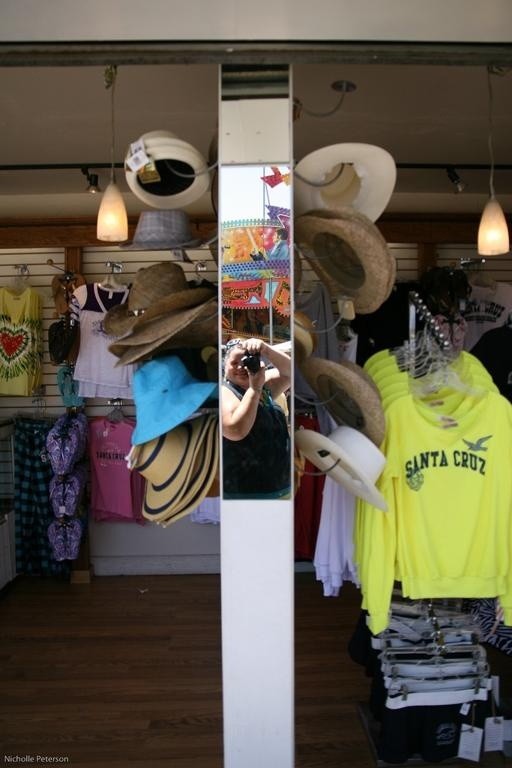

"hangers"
[391,308,482,400]
[101,262,122,290]
[106,399,124,423]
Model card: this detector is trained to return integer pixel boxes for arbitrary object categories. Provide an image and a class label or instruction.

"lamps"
[444,166,467,191]
[79,63,132,243]
[476,63,510,256]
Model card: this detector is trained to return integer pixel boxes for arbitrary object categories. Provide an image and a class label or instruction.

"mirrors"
[216,61,294,500]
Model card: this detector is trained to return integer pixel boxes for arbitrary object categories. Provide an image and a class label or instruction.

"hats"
[295,425,392,514]
[124,128,211,210]
[101,260,222,369]
[299,355,386,450]
[294,141,399,315]
[123,357,220,528]
[118,210,202,251]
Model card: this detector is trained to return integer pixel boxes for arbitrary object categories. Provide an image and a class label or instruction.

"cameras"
[240,349,261,374]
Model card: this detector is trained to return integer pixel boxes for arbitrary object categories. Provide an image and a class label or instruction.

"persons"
[220,336,291,501]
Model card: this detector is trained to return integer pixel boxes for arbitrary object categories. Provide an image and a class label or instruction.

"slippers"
[47,413,89,477]
[48,318,80,366]
[48,516,82,561]
[51,273,89,314]
[56,364,86,409]
[49,474,81,519]
